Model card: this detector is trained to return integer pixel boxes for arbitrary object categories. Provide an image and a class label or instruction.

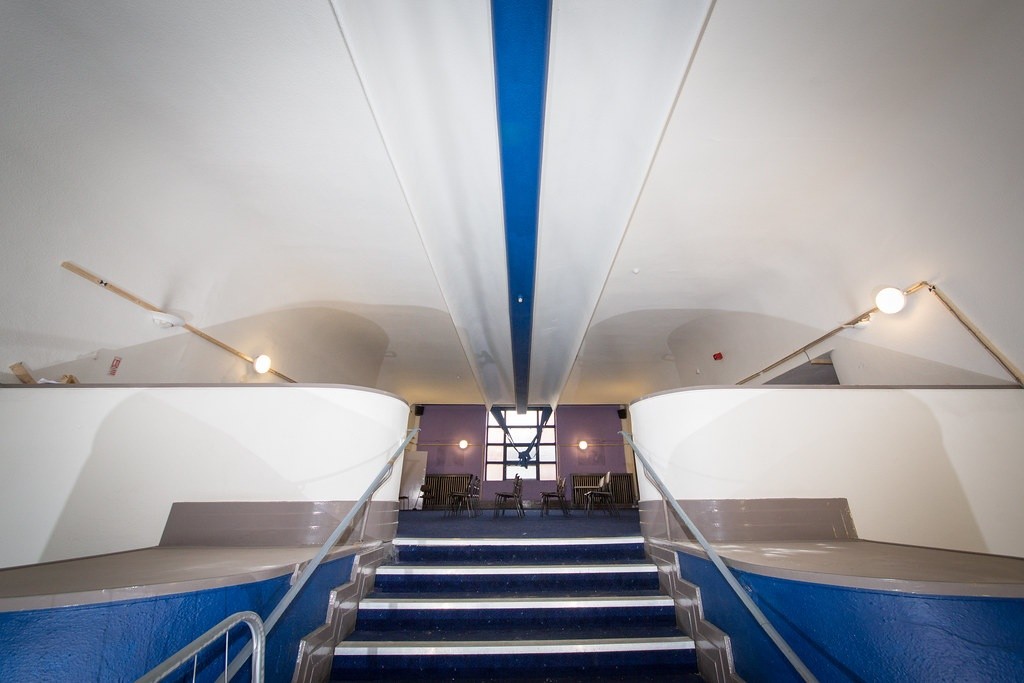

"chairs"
[398,472,622,518]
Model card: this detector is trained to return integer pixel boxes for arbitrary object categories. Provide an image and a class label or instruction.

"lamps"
[254,354,271,373]
[876,287,905,315]
[148,310,186,328]
